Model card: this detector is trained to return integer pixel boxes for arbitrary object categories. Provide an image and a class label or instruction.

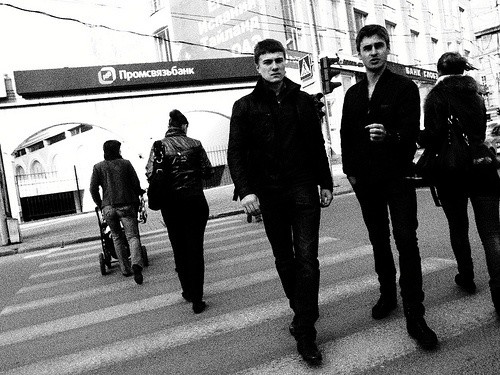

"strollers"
[95,201,150,275]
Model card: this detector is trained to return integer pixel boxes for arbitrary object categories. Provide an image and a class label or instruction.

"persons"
[340,24,440,351]
[90,140,146,286]
[416,52,500,326]
[145,109,214,315]
[227,39,334,367]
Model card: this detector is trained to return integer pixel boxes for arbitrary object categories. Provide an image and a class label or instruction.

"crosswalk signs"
[298,54,311,78]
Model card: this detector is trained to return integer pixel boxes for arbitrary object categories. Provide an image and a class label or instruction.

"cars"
[485,123,500,156]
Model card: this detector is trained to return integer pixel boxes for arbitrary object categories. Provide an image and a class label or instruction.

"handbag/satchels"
[200,165,225,188]
[147,170,178,209]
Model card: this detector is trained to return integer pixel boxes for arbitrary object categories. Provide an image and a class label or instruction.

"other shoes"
[123,269,133,277]
[406,315,438,352]
[289,322,323,365]
[182,291,206,314]
[132,264,143,285]
[454,273,477,294]
[371,297,398,320]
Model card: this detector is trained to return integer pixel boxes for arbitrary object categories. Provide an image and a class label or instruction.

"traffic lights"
[315,92,326,120]
[328,57,343,90]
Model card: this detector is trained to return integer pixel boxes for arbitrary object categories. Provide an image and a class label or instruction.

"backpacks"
[422,114,475,182]
